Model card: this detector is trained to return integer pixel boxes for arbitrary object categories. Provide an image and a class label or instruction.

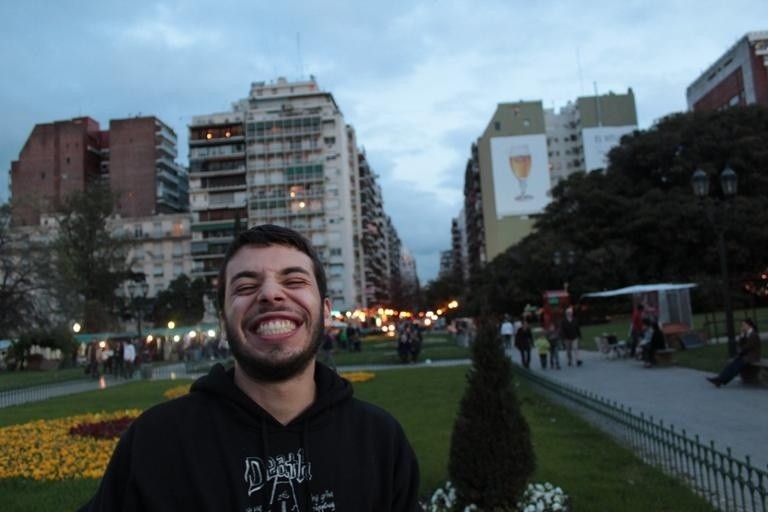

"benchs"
[656,347,677,366]
[739,358,768,387]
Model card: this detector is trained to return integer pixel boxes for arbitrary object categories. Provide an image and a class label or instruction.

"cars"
[355,317,445,337]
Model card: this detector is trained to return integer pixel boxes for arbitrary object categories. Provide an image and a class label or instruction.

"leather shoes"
[706,376,724,388]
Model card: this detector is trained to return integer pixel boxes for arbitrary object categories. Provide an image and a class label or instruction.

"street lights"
[687,163,741,362]
[125,279,151,366]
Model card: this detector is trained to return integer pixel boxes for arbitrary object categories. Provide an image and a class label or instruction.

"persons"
[323,317,423,365]
[83,327,230,381]
[447,302,667,368]
[705,318,761,389]
[95,223,422,510]
